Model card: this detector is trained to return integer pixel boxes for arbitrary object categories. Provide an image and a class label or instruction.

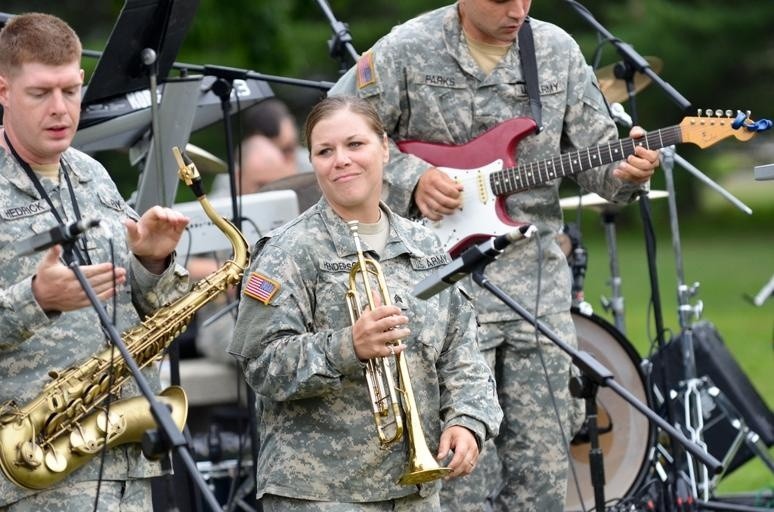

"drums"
[566,305,658,512]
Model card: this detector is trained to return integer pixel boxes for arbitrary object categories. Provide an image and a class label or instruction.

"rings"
[470,461,476,468]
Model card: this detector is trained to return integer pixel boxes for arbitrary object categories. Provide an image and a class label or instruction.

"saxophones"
[1,146,251,489]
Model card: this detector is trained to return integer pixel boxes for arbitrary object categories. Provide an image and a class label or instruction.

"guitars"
[399,111,772,252]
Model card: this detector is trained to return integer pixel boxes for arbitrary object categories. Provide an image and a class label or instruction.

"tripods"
[650,140,774,507]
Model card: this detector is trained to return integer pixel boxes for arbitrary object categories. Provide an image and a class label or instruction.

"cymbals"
[184,141,229,177]
[594,55,665,111]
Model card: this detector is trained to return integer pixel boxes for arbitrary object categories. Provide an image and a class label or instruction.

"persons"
[1,14,187,512]
[184,133,291,368]
[228,92,504,512]
[321,1,660,512]
[236,98,313,172]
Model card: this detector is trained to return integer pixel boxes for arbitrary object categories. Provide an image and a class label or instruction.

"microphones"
[12,213,101,256]
[413,227,524,300]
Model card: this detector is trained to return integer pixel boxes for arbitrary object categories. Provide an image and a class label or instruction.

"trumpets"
[344,220,452,482]
